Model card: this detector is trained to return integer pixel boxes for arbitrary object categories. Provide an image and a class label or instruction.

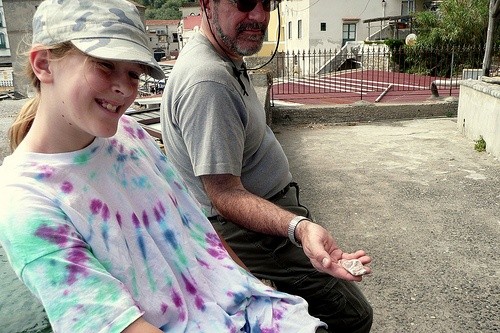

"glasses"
[237,0,279,12]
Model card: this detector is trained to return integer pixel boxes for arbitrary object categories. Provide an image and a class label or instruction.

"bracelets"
[288,215,310,248]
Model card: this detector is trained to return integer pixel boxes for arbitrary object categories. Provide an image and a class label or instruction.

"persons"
[160,0,377,333]
[0,0,321,333]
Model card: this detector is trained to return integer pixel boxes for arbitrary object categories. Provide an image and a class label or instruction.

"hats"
[33,0,166,81]
